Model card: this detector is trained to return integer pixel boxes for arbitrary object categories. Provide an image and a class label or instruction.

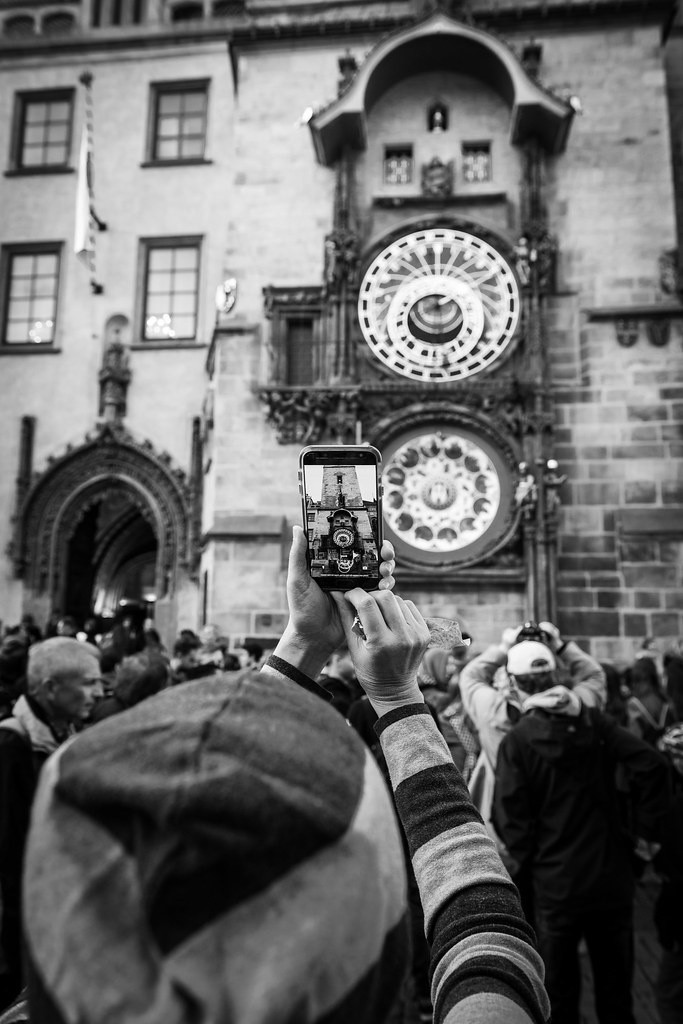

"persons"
[0,602,271,1018]
[0,527,551,1024]
[363,549,375,563]
[314,622,683,1024]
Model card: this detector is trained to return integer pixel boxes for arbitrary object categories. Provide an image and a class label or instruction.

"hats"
[22,668,409,1024]
[507,640,557,675]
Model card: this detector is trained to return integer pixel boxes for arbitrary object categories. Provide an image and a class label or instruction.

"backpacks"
[449,714,481,785]
[633,697,669,749]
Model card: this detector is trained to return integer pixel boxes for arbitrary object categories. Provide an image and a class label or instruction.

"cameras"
[515,623,551,645]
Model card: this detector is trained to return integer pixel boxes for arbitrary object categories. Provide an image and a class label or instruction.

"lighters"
[350,615,367,641]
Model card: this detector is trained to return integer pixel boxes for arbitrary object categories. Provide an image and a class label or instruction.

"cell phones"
[297,445,384,593]
